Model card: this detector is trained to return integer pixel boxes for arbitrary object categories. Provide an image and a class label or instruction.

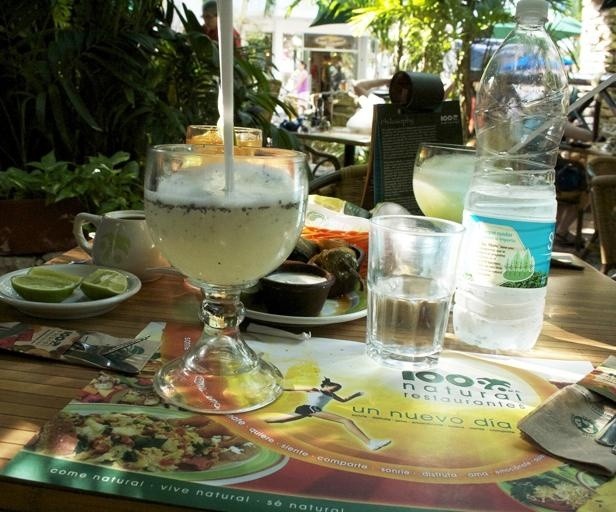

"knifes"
[61,351,140,378]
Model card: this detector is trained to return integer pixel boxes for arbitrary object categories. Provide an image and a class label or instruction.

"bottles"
[453,0,571,355]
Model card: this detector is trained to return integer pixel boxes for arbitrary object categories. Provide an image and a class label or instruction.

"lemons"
[27,267,83,289]
[80,268,127,299]
[11,274,75,303]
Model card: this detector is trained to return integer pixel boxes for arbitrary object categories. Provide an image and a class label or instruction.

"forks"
[67,335,151,356]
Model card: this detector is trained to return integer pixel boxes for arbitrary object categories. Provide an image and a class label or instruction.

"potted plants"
[0,148,143,256]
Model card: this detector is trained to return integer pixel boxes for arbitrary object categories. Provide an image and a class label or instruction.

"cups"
[260,260,336,316]
[364,215,465,373]
[412,141,477,223]
[73,209,171,284]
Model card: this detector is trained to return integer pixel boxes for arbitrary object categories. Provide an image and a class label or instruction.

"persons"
[197,0,240,49]
[321,51,344,115]
[309,58,321,111]
[261,374,395,453]
[286,59,310,118]
[508,85,594,248]
[459,78,525,150]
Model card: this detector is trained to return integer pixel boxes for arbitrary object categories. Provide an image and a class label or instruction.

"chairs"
[256,79,373,210]
[581,155,616,275]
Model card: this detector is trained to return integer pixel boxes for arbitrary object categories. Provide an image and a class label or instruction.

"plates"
[246,289,368,327]
[0,264,143,320]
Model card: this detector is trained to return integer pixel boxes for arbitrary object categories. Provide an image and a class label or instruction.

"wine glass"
[144,143,310,415]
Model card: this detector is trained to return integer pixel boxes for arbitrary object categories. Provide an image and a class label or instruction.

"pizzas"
[23,413,260,471]
